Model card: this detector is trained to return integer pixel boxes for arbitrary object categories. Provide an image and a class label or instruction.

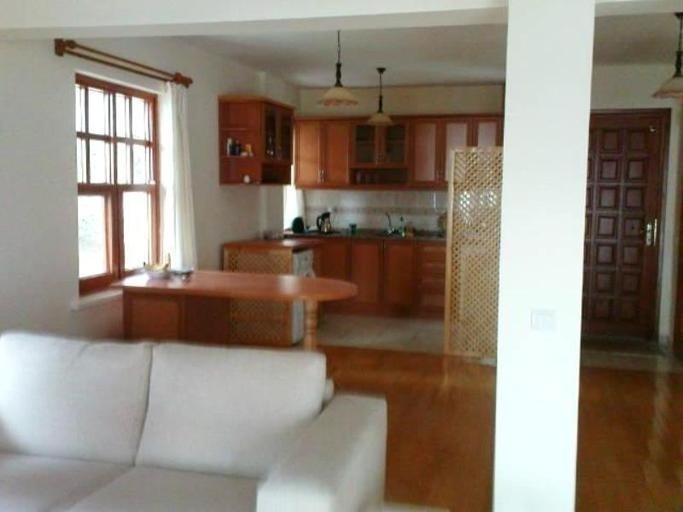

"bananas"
[144,262,169,272]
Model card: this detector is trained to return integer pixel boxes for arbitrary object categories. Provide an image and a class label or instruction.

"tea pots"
[316,212,331,234]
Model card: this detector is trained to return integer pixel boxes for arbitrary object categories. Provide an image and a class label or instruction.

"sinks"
[375,233,396,237]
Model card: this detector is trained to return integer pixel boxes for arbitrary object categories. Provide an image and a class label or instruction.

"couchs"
[0,330,389,511]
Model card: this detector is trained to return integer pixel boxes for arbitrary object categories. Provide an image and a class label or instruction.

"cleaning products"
[399,216,406,238]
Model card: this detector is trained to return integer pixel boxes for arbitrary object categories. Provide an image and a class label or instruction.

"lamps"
[316,31,392,126]
[650,13,682,100]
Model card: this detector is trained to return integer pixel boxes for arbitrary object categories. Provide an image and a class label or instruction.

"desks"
[109,270,359,350]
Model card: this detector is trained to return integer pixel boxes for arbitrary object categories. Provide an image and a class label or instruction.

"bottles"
[226,137,240,156]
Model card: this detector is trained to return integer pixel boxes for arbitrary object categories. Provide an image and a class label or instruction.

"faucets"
[384,211,396,235]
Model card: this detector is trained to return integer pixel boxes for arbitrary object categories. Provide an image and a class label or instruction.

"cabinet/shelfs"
[319,242,349,312]
[349,118,411,169]
[417,243,445,315]
[351,243,416,312]
[216,102,293,185]
[411,118,471,188]
[472,117,500,148]
[294,120,351,190]
